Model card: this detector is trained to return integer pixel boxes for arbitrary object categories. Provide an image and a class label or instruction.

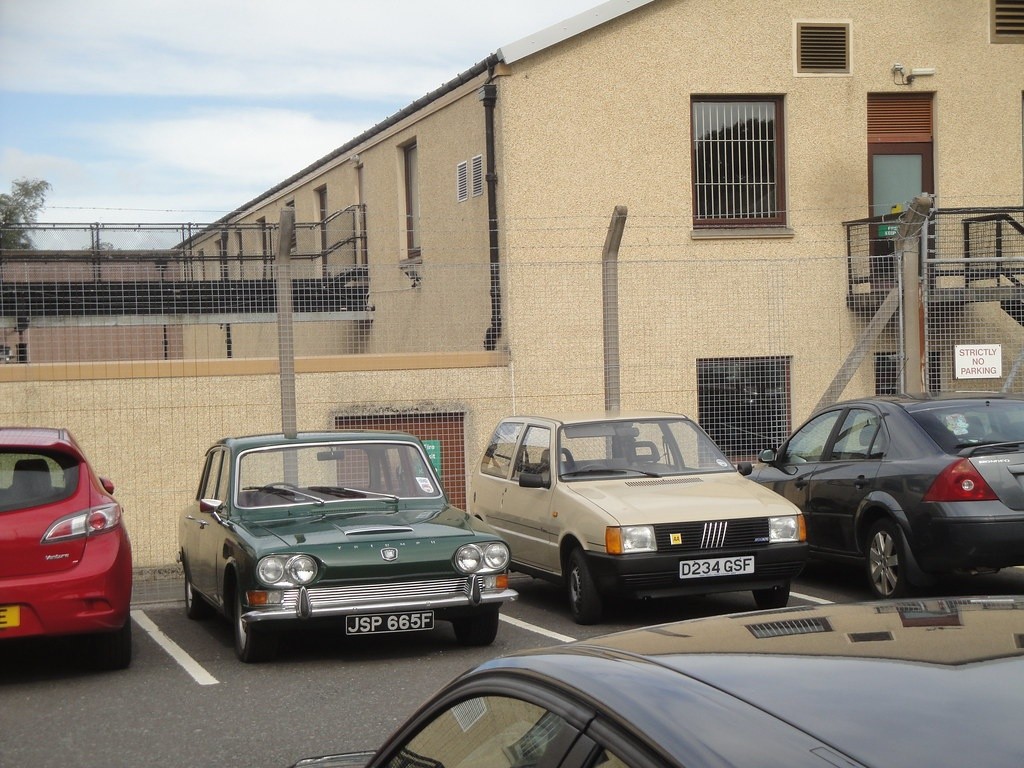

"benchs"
[529,458,637,473]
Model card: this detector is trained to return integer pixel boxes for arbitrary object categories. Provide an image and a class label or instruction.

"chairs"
[959,416,985,438]
[859,424,880,446]
[541,448,574,475]
[12,459,52,489]
[627,441,660,466]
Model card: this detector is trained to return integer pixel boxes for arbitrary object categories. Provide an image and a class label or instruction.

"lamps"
[911,68,934,76]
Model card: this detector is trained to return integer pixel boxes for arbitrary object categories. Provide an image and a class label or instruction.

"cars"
[177,429,519,664]
[749,393,1024,604]
[0,426,133,672]
[471,411,811,624]
[367,595,1024,768]
